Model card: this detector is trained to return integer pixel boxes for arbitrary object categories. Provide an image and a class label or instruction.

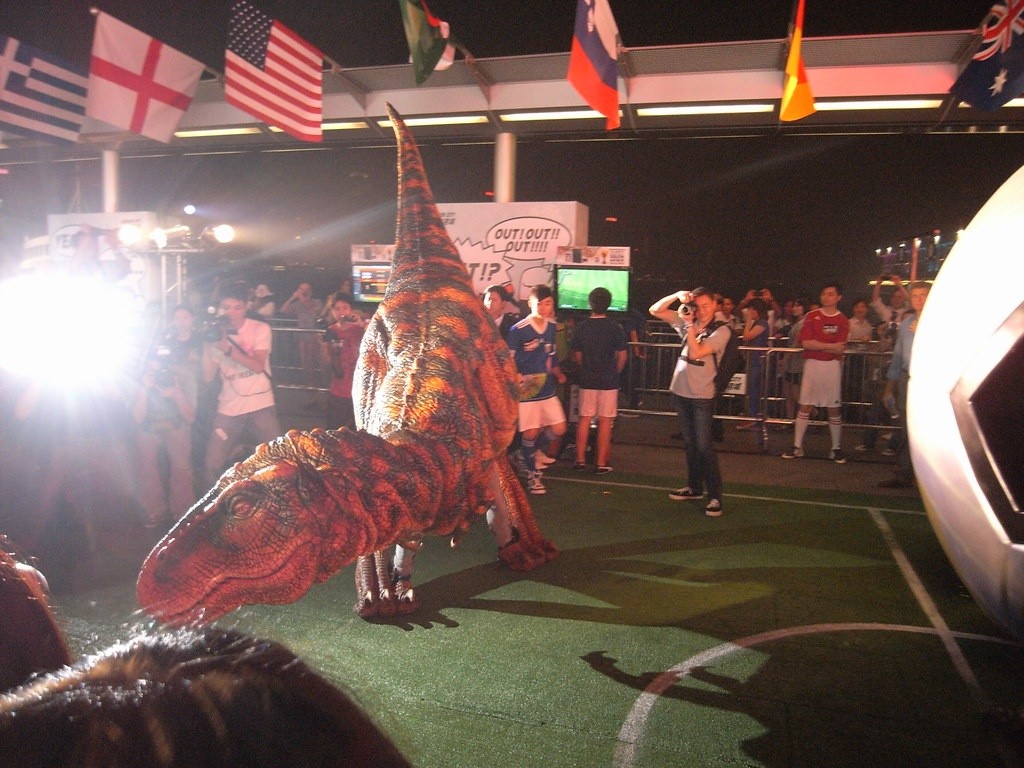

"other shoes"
[854,443,870,452]
[594,465,613,474]
[882,448,895,456]
[145,512,164,527]
[574,460,585,472]
[620,412,641,418]
[736,420,757,429]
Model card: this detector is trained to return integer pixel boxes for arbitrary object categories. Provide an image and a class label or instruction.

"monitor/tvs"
[553,263,633,321]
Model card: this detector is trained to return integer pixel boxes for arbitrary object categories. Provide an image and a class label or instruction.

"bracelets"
[684,323,694,328]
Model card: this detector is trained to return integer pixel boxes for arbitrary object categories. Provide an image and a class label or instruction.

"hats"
[256,285,273,297]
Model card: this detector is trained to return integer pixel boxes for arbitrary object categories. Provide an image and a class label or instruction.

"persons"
[480,284,645,494]
[672,273,932,464]
[135,278,371,528]
[649,287,731,516]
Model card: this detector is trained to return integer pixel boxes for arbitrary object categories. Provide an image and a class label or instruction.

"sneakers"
[782,446,805,459]
[527,472,546,495]
[705,496,722,515]
[669,487,704,500]
[829,448,846,464]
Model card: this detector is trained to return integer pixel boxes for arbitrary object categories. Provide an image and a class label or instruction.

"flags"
[779,0,817,121]
[223,0,325,144]
[401,0,453,86]
[948,0,1024,111]
[84,12,206,144]
[0,34,87,146]
[564,0,627,131]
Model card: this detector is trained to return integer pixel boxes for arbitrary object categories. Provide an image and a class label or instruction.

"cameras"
[681,299,697,315]
[316,314,338,343]
[200,302,231,341]
[342,314,357,323]
[883,276,891,280]
[151,345,174,386]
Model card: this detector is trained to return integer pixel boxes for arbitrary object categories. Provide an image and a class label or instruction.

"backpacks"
[706,320,744,392]
